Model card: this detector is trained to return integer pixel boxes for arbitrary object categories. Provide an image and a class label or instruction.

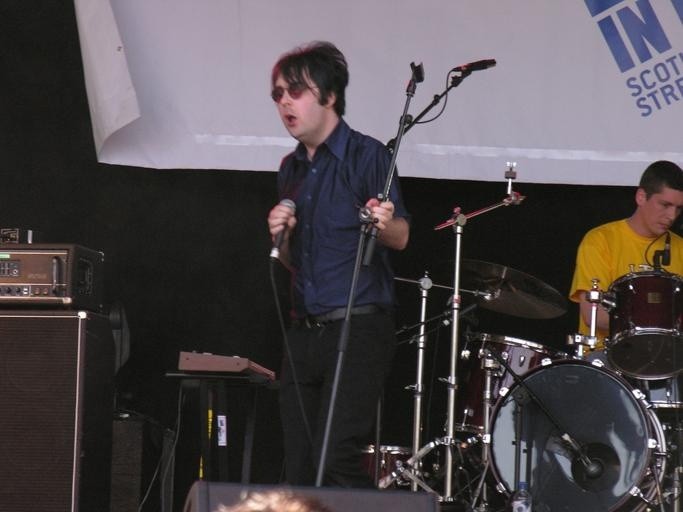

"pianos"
[179,349,276,381]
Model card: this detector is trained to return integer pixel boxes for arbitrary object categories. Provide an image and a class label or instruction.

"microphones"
[270,198,296,260]
[452,59,496,71]
[662,235,670,265]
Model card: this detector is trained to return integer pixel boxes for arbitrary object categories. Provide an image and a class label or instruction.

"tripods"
[376,217,514,503]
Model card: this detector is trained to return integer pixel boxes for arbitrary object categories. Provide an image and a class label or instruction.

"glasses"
[271,80,318,102]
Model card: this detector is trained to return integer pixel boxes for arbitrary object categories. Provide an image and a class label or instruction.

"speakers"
[0,311,116,512]
[183,481,438,512]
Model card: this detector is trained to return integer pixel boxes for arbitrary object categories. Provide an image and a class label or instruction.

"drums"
[445,330,570,434]
[484,358,666,512]
[360,443,413,487]
[606,270,683,380]
[624,376,683,410]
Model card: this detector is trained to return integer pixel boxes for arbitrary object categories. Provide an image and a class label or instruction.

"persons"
[267,39,414,489]
[214,489,330,512]
[568,161,683,358]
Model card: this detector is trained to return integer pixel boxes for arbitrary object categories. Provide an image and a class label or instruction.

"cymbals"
[429,257,570,320]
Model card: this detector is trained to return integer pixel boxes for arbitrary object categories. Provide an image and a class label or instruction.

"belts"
[305,302,382,329]
